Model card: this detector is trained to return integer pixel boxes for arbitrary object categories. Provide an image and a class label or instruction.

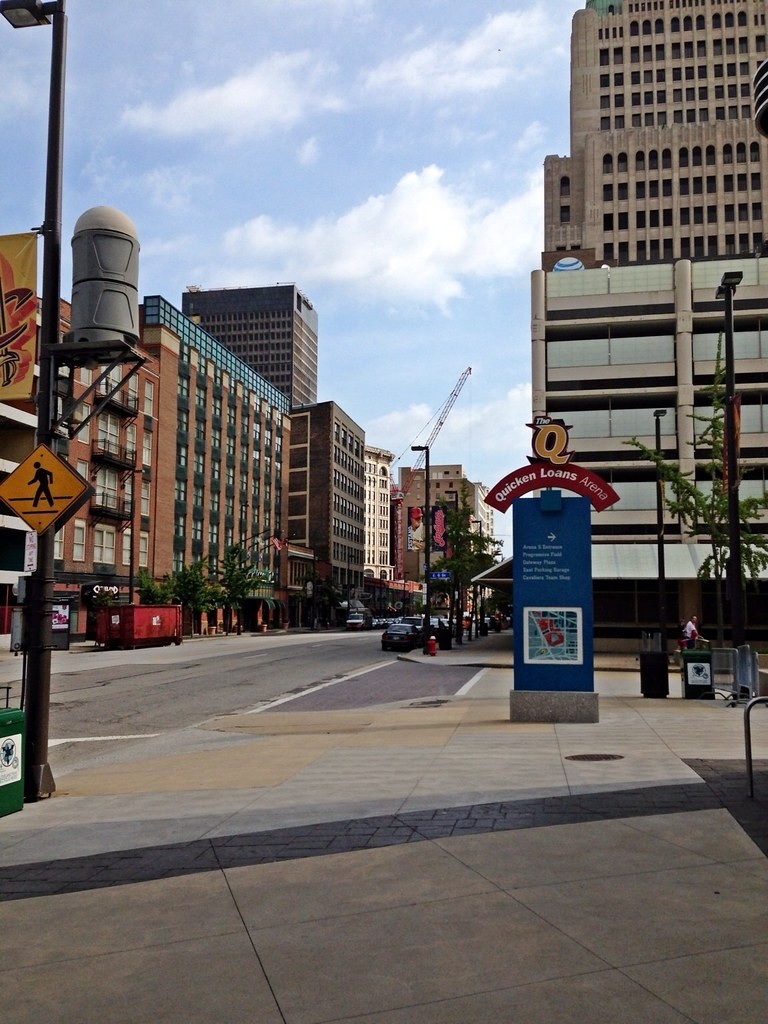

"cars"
[373,617,402,629]
[381,624,420,652]
[431,617,472,646]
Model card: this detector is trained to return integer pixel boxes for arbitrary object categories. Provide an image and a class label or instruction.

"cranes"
[391,367,472,581]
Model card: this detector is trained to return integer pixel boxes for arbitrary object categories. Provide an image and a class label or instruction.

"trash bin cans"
[639,651,670,698]
[0,707,26,818]
[480,623,488,636]
[438,626,451,649]
[680,638,716,700]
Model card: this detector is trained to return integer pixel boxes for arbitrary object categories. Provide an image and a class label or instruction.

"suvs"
[399,615,425,644]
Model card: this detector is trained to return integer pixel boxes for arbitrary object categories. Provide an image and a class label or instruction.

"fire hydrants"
[429,636,438,657]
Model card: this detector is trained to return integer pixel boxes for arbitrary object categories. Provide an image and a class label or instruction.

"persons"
[683,615,702,650]
[676,615,687,654]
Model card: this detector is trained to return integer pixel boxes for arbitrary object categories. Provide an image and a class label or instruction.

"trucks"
[346,607,371,632]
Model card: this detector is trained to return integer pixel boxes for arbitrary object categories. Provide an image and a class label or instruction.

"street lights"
[472,520,485,637]
[0,0,67,803]
[347,555,356,620]
[444,490,463,644]
[411,445,431,655]
[653,409,667,665]
